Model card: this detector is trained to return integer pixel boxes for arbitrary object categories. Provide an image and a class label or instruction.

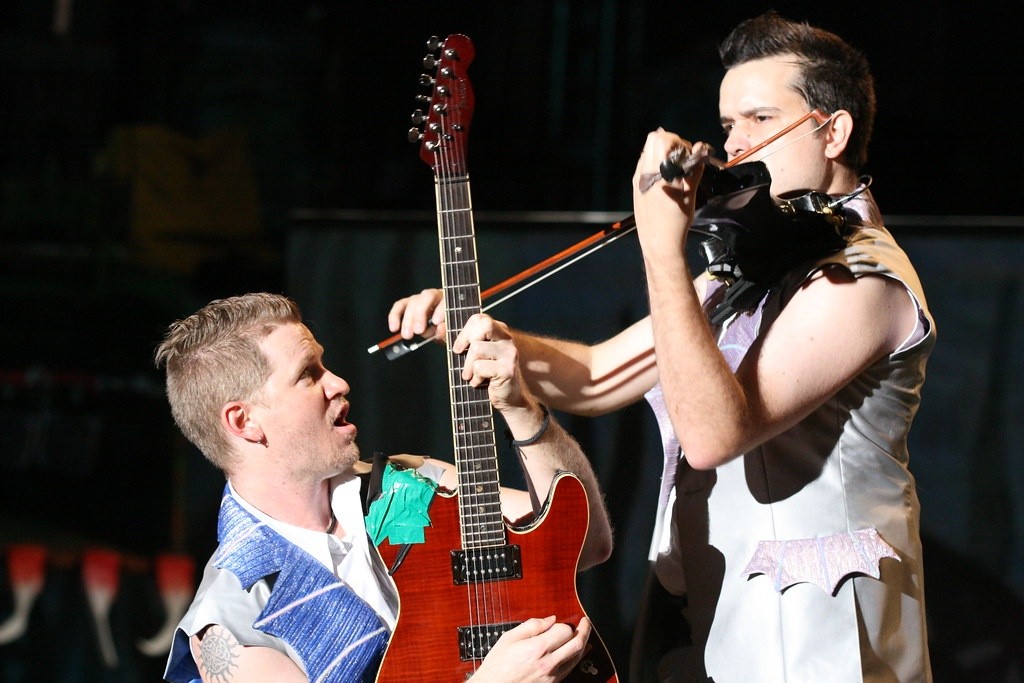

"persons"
[389,15,936,683]
[156,294,612,682]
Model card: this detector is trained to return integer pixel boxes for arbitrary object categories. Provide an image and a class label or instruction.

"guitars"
[364,30,623,683]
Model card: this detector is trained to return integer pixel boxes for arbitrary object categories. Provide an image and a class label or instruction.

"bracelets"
[511,403,550,446]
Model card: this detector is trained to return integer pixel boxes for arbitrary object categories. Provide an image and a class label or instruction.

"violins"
[642,140,850,315]
